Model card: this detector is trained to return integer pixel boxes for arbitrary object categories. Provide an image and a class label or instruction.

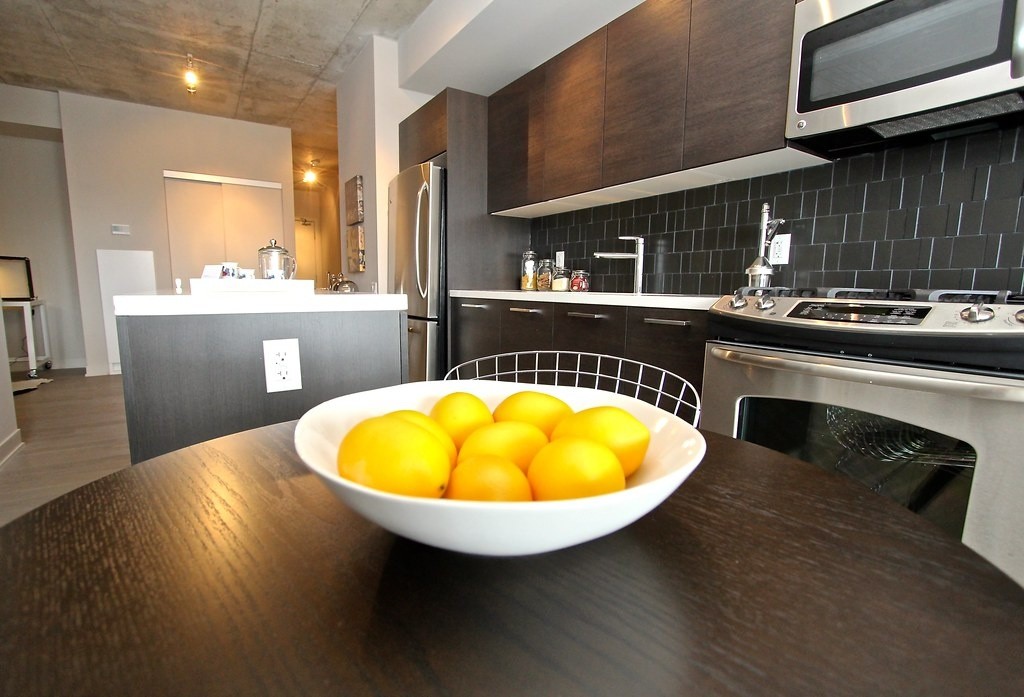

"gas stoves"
[706,286,1023,373]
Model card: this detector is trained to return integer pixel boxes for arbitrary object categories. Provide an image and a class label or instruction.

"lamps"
[186,54,196,94]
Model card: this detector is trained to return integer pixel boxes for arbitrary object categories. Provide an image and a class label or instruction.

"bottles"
[571,270,590,291]
[537,259,554,290]
[551,267,571,292]
[520,250,537,290]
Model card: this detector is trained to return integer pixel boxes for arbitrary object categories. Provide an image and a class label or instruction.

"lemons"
[337,391,650,502]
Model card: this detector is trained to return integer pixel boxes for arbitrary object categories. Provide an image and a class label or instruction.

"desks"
[0,419,1024,697]
[2,300,52,378]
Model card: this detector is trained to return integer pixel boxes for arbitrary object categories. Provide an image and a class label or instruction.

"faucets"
[593,235,644,293]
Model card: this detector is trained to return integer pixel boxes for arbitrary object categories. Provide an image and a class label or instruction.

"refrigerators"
[386,164,447,384]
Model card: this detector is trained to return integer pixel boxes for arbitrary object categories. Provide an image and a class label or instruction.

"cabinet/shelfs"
[399,0,796,429]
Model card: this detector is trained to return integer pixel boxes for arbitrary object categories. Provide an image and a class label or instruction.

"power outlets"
[263,338,303,393]
[769,234,791,265]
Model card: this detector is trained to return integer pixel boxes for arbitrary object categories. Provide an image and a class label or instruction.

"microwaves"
[786,3,1024,163]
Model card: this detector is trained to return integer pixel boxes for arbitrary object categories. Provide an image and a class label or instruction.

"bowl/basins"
[293,382,704,555]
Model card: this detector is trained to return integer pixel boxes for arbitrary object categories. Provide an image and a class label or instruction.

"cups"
[258,241,298,281]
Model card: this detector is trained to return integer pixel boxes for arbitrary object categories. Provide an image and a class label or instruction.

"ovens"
[696,342,1022,582]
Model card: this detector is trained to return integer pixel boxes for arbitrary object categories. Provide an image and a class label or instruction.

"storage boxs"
[189,279,315,296]
